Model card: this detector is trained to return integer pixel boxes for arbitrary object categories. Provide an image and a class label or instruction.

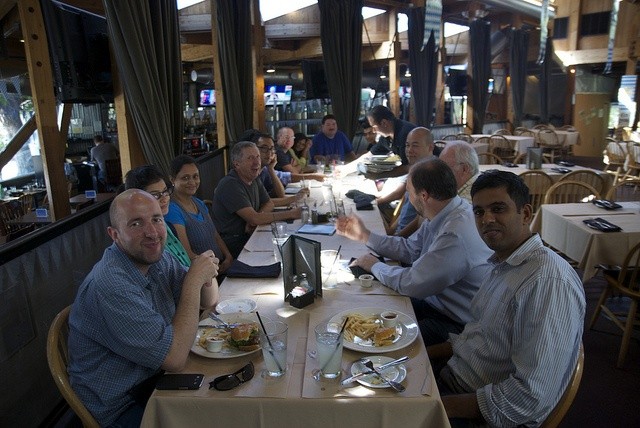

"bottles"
[301,206,309,225]
[311,209,319,225]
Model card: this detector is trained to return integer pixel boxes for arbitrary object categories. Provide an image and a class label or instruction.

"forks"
[354,359,406,392]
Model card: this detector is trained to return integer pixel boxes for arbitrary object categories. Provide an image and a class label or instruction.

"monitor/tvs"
[299,56,330,101]
[486,75,495,95]
[263,80,295,106]
[448,68,467,97]
[194,85,217,109]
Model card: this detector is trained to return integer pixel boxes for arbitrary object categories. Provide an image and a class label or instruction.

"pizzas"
[201,327,229,348]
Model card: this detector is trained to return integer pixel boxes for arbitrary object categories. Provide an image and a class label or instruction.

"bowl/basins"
[359,274,374,287]
[380,311,398,329]
[206,337,224,352]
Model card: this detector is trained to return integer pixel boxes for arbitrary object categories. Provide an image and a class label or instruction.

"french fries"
[339,310,376,343]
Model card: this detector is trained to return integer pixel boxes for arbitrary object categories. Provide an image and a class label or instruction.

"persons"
[274,127,317,175]
[67,188,219,427]
[388,141,480,234]
[310,115,357,162]
[114,165,175,231]
[250,135,285,198]
[360,127,485,234]
[332,105,450,158]
[212,141,308,254]
[363,125,376,145]
[292,132,313,160]
[435,171,586,428]
[396,140,485,234]
[91,134,122,189]
[162,156,234,274]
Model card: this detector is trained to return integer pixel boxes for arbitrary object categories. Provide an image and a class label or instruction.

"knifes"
[341,356,408,385]
[198,323,243,330]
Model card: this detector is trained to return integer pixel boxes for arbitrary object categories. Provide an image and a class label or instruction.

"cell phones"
[152,370,205,392]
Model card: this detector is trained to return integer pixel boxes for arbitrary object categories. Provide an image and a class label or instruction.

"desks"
[607,141,640,187]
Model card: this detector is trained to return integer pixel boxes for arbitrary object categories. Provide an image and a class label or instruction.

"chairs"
[492,129,512,136]
[607,180,640,202]
[584,240,640,369]
[199,200,215,224]
[434,141,448,157]
[455,133,474,143]
[535,129,563,164]
[102,159,122,193]
[533,125,549,131]
[486,134,524,164]
[443,135,458,140]
[551,124,576,160]
[620,140,640,196]
[0,201,22,234]
[606,177,640,202]
[513,153,550,164]
[599,136,638,195]
[13,193,36,229]
[554,170,606,202]
[474,137,496,164]
[513,171,556,228]
[475,152,504,164]
[38,180,70,209]
[439,331,584,428]
[44,303,143,428]
[544,180,615,303]
[34,194,48,227]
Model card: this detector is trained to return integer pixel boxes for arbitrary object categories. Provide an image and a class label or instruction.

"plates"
[215,298,257,313]
[327,307,419,353]
[352,355,406,391]
[190,314,263,360]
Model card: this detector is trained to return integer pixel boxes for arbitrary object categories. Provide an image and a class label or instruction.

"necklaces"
[172,192,198,214]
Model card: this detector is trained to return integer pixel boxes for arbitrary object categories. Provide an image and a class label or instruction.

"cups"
[316,160,323,175]
[272,235,290,268]
[271,221,287,238]
[322,184,335,202]
[259,320,288,376]
[300,179,310,190]
[320,249,339,291]
[315,323,343,379]
[332,199,345,219]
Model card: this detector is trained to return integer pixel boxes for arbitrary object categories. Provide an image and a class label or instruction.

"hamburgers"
[228,325,260,350]
[373,327,397,344]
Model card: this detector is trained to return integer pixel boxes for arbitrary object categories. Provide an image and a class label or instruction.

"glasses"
[208,360,255,391]
[258,145,278,154]
[151,187,173,200]
